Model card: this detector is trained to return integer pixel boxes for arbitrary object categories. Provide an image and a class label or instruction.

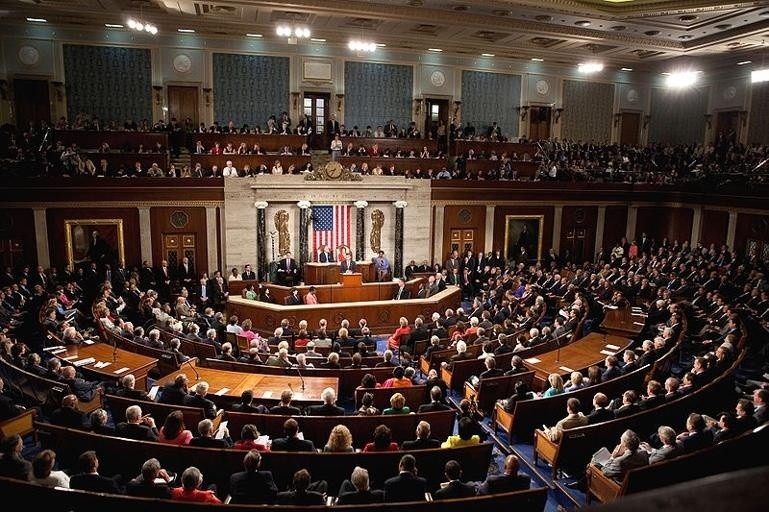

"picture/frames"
[64,218,125,270]
[503,215,544,263]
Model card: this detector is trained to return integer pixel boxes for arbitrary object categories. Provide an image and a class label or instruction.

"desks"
[227,272,463,332]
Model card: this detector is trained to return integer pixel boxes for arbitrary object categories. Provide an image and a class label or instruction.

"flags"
[313,206,353,263]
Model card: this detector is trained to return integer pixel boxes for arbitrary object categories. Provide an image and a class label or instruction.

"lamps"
[127,4,160,38]
[277,13,310,38]
[348,24,376,52]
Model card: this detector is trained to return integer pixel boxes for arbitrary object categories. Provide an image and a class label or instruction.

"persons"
[0,232,769,510]
[337,246,351,263]
[516,225,530,254]
[374,250,390,281]
[0,109,769,191]
[84,231,109,264]
[276,252,301,286]
[317,245,333,263]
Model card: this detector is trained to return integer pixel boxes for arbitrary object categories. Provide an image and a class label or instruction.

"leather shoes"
[564,481,586,493]
